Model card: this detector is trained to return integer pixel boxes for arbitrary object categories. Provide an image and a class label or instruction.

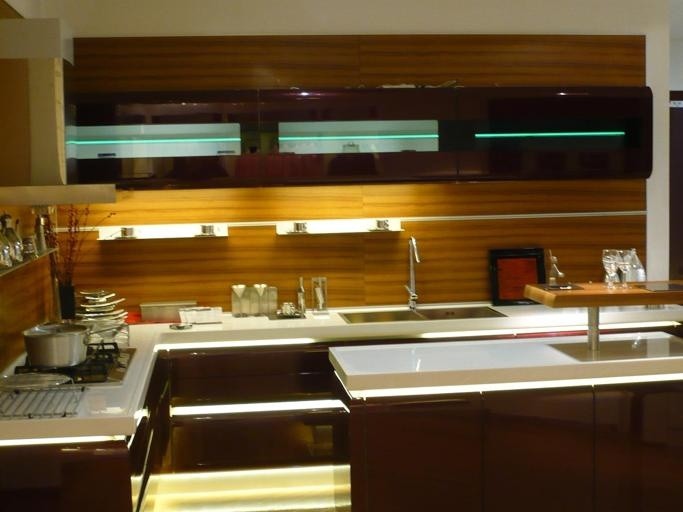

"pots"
[24,323,129,366]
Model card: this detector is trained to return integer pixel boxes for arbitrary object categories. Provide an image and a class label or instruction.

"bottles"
[297,276,305,313]
[0,213,48,269]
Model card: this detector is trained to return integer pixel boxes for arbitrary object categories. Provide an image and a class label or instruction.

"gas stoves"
[14,339,137,387]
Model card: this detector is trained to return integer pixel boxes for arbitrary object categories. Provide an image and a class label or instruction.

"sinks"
[404,235,422,311]
[339,310,428,323]
[414,306,507,320]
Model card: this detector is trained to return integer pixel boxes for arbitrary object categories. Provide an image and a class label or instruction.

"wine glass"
[231,283,266,318]
[602,249,633,288]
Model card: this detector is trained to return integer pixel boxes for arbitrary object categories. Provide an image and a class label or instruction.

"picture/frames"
[488,248,546,305]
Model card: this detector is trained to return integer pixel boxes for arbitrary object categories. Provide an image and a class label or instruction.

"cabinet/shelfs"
[117,342,349,512]
[347,380,683,512]
